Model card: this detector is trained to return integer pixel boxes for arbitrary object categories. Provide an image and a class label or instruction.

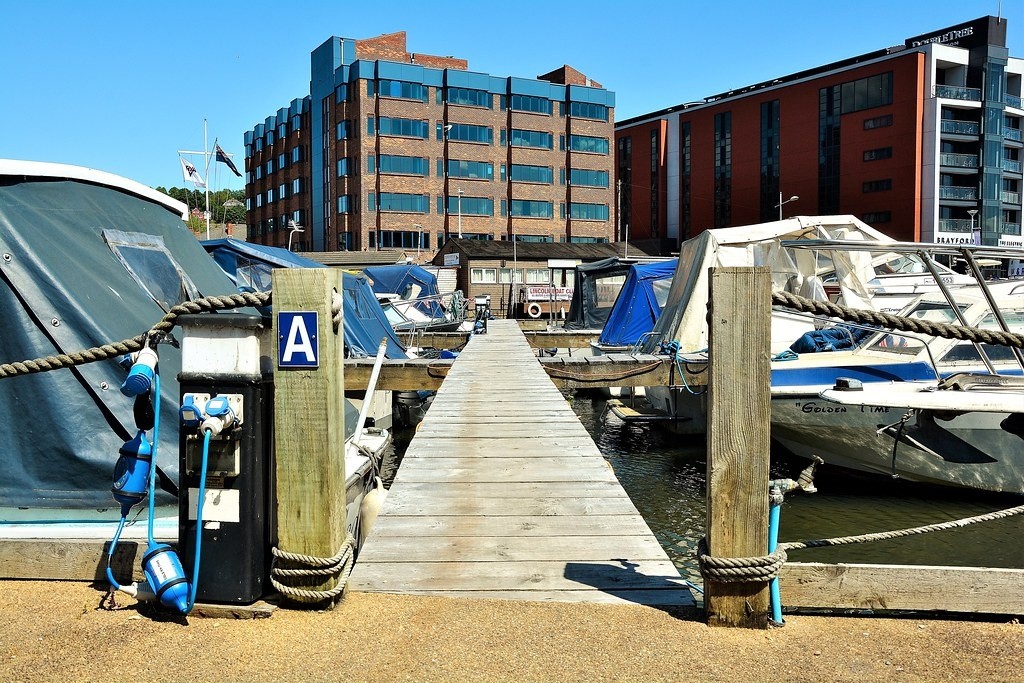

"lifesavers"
[528,302,542,318]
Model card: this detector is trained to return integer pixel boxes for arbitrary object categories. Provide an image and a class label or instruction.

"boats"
[536,209,1024,500]
[0,157,462,538]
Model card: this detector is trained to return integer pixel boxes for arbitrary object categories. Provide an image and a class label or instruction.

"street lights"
[780,196,799,220]
[967,209,978,244]
[457,191,464,238]
